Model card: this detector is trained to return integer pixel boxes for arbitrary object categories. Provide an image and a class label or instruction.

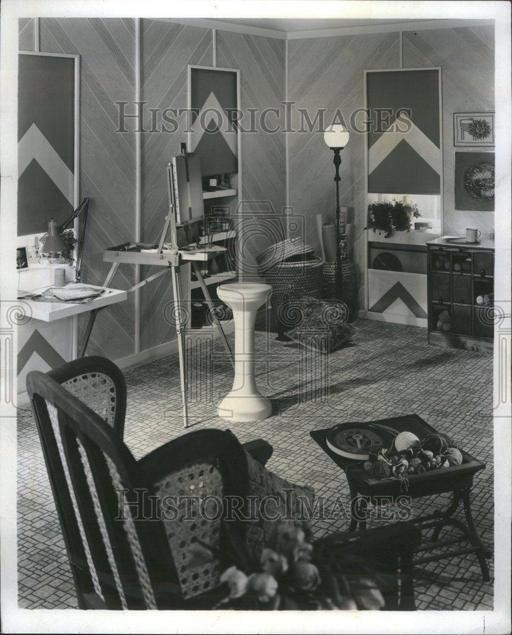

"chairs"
[26,356,421,609]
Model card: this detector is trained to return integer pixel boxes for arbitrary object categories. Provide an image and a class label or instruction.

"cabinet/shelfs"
[191,189,239,346]
[427,242,494,343]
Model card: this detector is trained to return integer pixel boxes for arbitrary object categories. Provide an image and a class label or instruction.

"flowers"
[196,518,386,610]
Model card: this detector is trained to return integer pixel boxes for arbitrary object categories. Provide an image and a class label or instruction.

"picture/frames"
[452,112,494,148]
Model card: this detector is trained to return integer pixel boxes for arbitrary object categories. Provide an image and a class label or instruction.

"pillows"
[287,302,353,354]
[226,428,314,530]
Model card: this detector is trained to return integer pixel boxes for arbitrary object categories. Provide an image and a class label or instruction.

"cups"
[55,268,65,286]
[466,228,481,243]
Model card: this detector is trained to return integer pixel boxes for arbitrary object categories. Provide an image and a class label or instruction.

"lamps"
[324,123,349,301]
[37,198,89,283]
